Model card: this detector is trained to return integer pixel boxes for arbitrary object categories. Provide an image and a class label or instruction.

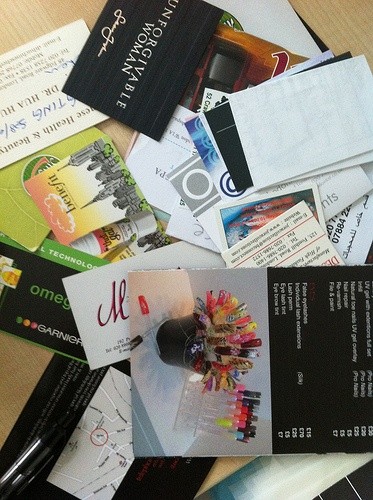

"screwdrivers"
[130,317,170,351]
[138,295,161,355]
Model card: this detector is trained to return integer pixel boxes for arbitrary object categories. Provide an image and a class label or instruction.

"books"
[127,262,373,459]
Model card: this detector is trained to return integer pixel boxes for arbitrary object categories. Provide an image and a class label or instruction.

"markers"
[0,409,77,500]
